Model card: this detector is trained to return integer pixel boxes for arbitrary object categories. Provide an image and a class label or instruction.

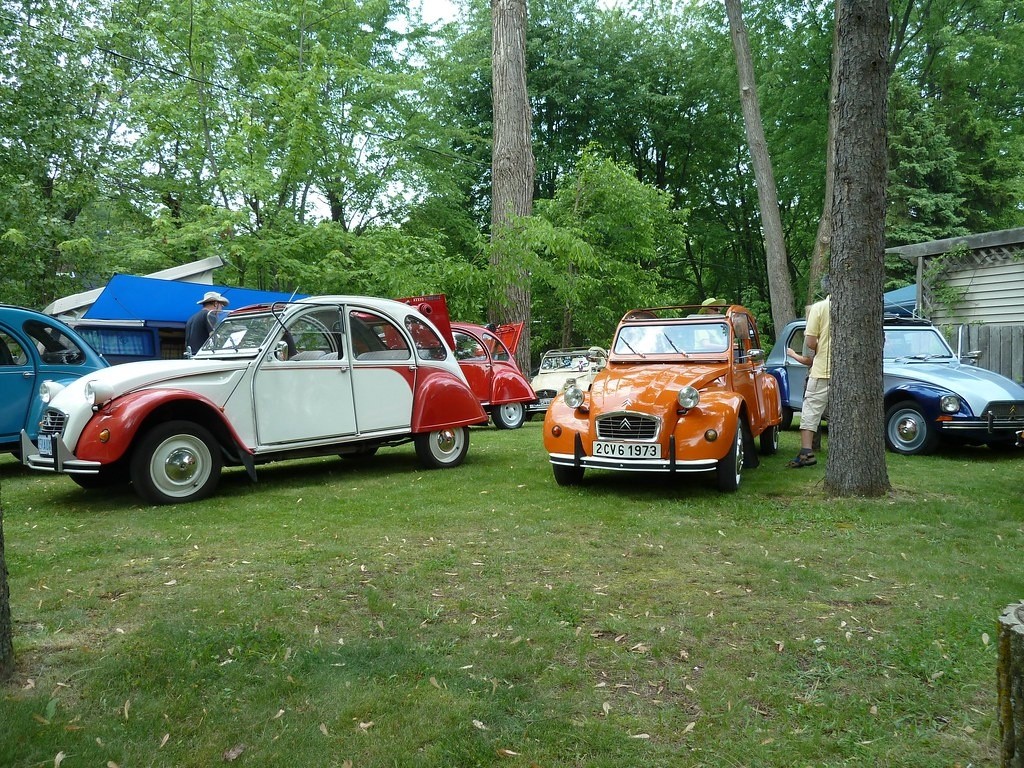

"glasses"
[711,308,721,312]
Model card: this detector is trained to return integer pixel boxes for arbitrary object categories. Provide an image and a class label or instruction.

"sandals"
[785,453,817,468]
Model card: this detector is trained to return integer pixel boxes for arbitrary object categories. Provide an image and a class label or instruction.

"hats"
[197,292,229,306]
[698,298,726,315]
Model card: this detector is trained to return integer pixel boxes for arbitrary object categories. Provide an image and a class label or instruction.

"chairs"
[621,339,654,353]
[288,349,432,360]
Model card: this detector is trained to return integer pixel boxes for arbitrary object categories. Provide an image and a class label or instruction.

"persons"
[786,276,831,467]
[185,291,230,356]
[698,298,727,313]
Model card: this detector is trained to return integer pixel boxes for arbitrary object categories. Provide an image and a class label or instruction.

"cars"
[543,304,785,493]
[378,320,538,431]
[0,302,112,462]
[523,346,608,422]
[18,294,490,505]
[764,315,1024,457]
